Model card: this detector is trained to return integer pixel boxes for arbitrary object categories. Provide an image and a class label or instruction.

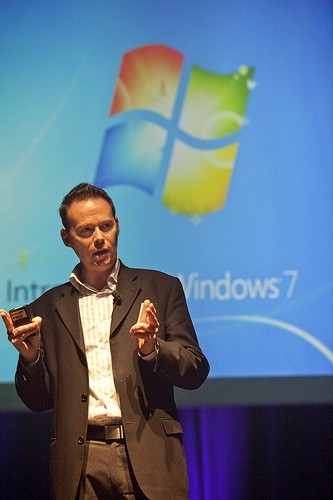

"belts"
[86,424,125,441]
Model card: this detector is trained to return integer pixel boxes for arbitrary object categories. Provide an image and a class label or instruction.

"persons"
[0,181,210,500]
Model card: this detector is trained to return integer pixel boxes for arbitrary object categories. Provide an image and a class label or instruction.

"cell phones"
[9,305,37,339]
